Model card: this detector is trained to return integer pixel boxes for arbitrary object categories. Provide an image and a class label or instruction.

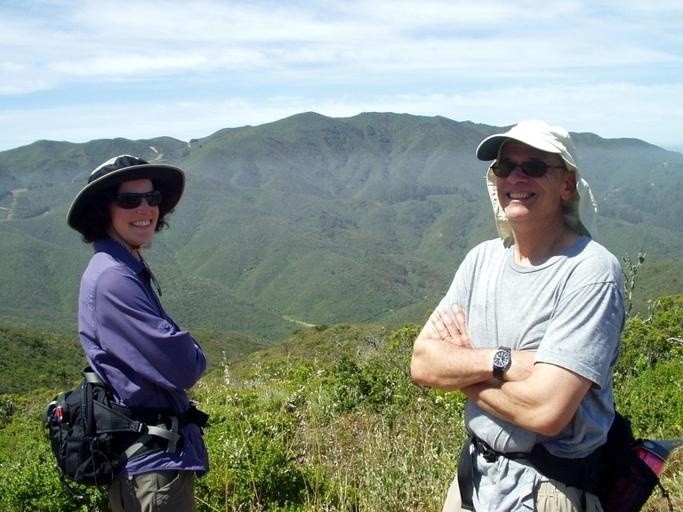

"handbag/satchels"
[47,367,180,486]
[602,411,658,511]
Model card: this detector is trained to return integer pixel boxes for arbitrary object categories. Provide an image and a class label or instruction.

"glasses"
[490,160,563,176]
[111,189,162,209]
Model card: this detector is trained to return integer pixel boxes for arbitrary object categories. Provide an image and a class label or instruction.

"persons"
[64,150,213,512]
[406,118,630,512]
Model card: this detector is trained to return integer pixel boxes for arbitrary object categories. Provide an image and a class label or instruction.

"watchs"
[490,345,513,383]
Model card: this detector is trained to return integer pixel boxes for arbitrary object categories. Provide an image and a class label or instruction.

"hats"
[66,154,185,235]
[476,119,578,170]
